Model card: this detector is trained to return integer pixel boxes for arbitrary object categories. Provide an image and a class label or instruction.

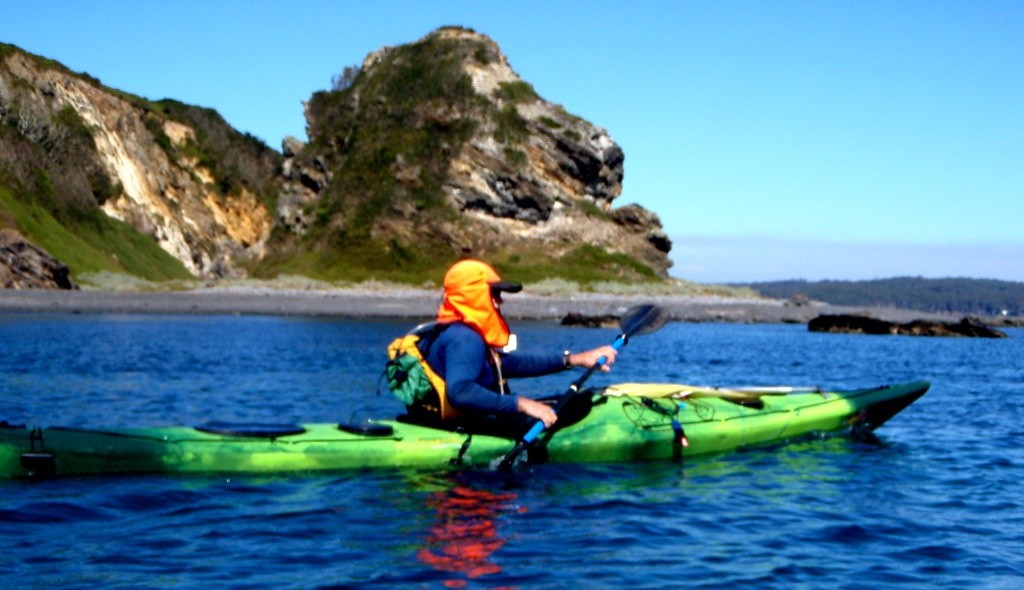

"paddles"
[496,303,670,481]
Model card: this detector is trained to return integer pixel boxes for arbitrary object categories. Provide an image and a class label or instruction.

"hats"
[489,281,523,294]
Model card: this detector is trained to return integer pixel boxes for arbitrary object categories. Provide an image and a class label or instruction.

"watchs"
[560,350,571,366]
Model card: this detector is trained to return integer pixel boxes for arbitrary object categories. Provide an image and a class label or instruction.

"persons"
[406,259,619,428]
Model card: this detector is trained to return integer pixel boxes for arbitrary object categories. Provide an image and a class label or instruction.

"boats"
[1,374,933,482]
